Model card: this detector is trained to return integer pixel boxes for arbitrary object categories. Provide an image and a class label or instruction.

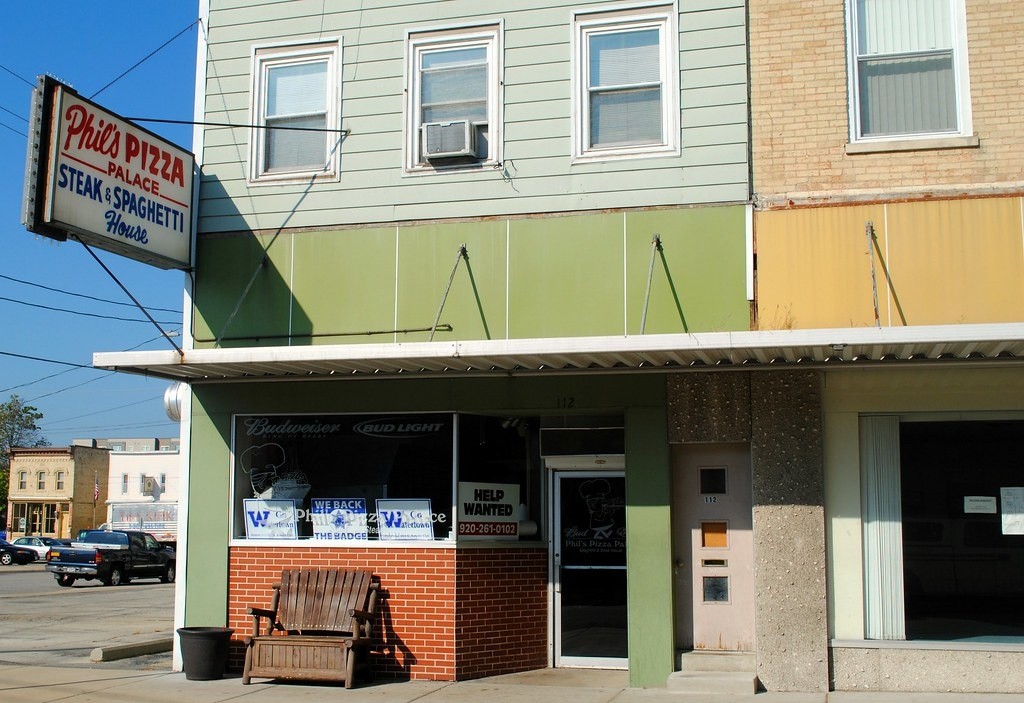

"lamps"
[502,417,513,429]
[511,418,520,427]
[54,511,59,519]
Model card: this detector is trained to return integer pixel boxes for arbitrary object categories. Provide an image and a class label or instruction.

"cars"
[8,536,64,561]
[0,536,40,566]
[76,528,106,542]
[52,538,77,547]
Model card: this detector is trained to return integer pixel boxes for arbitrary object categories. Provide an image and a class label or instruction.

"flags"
[94,470,103,502]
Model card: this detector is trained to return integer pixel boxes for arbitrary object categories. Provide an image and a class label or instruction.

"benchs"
[242,567,384,690]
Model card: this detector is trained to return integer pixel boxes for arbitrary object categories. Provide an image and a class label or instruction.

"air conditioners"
[421,121,476,160]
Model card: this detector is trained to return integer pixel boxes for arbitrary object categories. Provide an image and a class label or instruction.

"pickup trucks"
[44,530,176,586]
